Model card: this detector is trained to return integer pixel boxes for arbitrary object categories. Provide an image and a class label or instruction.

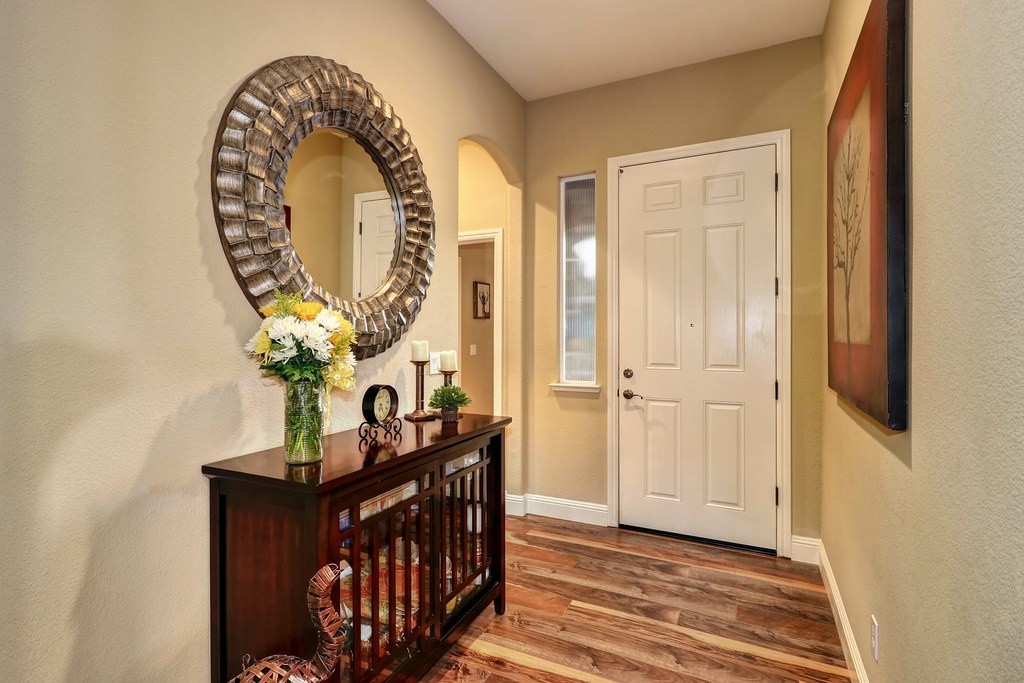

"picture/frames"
[473,281,490,319]
[825,0,911,432]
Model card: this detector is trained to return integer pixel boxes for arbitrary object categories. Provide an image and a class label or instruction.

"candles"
[439,350,457,370]
[412,340,428,361]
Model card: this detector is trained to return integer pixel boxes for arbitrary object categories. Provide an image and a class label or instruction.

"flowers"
[244,282,356,391]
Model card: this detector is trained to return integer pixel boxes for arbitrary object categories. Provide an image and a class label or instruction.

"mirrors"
[211,55,436,362]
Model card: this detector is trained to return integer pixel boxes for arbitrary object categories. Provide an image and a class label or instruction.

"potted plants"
[428,384,472,422]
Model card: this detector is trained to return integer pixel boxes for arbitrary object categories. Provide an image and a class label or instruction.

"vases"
[284,381,325,464]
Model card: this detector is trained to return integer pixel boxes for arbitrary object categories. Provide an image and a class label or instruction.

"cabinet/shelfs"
[199,411,512,683]
